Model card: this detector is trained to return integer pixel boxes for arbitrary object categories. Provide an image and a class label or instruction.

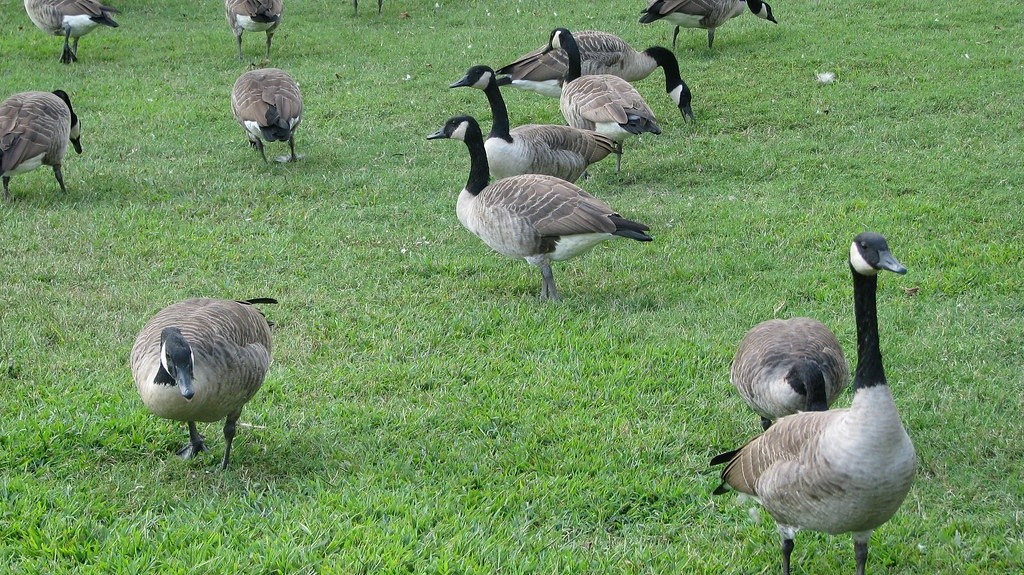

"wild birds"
[427,116,653,300]
[450,65,623,184]
[22,0,123,65]
[712,233,918,575]
[231,64,304,163]
[542,28,662,180]
[225,0,283,58]
[129,297,278,472]
[728,317,848,431]
[639,0,778,51]
[495,31,696,124]
[0,90,83,201]
